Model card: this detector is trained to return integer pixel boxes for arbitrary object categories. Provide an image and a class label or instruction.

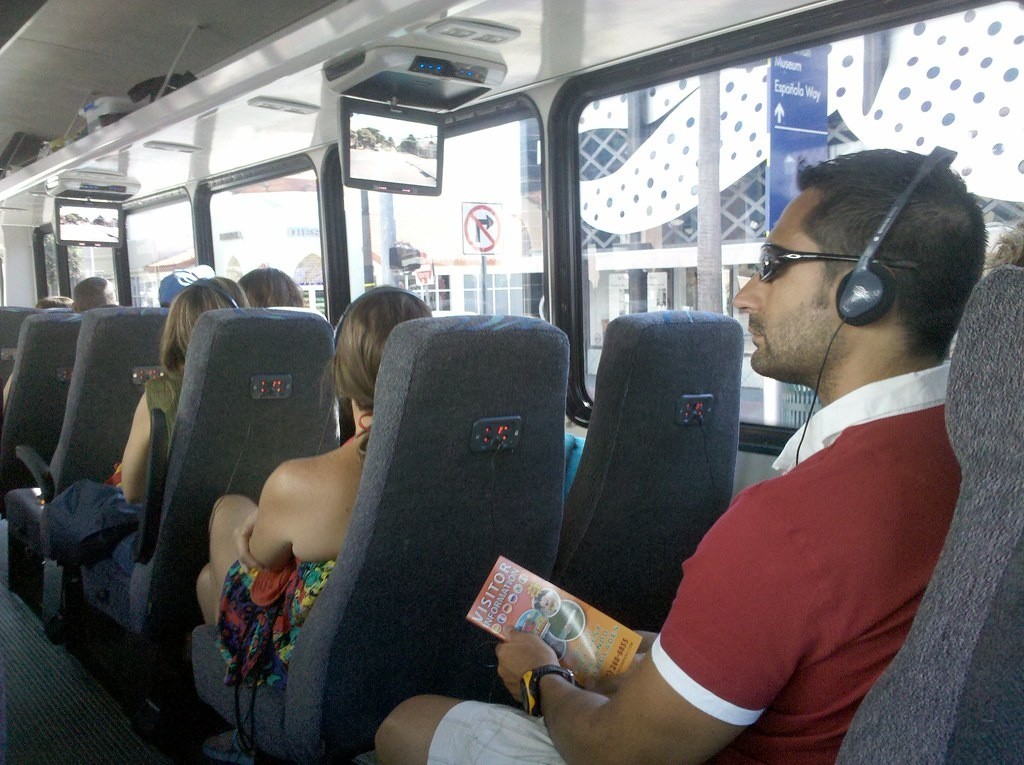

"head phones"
[835,146,958,327]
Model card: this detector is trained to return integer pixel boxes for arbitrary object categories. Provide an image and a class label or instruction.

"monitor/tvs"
[338,96,445,196]
[53,198,124,249]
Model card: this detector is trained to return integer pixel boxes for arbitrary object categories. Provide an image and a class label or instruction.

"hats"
[159,265,216,305]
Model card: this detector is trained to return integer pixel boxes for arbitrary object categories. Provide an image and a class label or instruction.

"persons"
[120,146,1024,765]
[35,296,73,308]
[2,277,120,414]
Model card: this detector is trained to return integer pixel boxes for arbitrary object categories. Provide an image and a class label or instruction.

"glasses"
[755,241,912,282]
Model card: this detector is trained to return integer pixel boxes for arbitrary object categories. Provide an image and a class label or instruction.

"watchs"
[520,665,575,718]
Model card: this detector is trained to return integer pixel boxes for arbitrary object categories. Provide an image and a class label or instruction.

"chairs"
[0,306,342,640]
[834,263,1024,765]
[191,311,743,765]
